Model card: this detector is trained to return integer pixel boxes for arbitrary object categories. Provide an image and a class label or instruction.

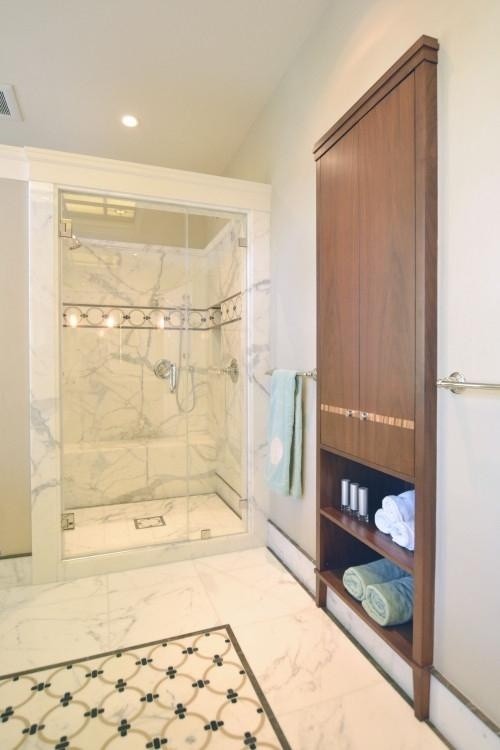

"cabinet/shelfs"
[315,63,439,668]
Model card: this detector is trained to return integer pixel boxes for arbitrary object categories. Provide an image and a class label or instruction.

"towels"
[264,367,304,498]
[342,557,410,601]
[373,488,415,551]
[362,577,413,627]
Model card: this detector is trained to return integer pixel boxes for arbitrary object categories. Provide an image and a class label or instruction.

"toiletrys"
[340,478,350,513]
[349,482,359,519]
[357,487,369,523]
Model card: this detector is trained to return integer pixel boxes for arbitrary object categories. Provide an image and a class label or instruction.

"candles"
[358,487,369,516]
[340,478,350,505]
[349,482,358,511]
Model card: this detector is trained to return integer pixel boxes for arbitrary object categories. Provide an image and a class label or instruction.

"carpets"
[0,624,292,749]
